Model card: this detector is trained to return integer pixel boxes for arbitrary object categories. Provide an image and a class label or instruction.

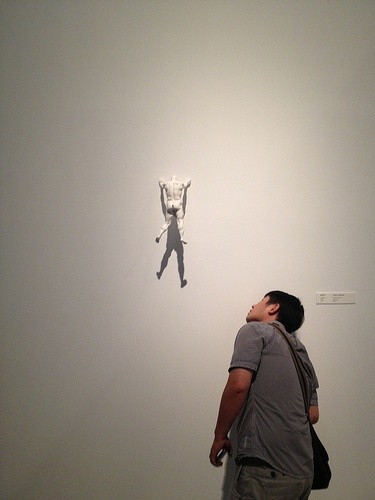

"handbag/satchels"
[309,420,331,491]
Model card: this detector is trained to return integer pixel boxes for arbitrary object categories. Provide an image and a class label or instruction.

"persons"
[155,175,192,245]
[208,290,320,500]
